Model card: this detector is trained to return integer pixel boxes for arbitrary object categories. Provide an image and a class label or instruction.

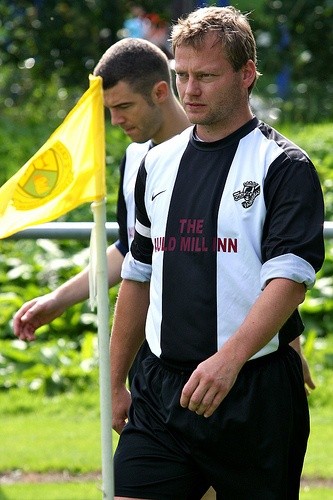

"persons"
[106,8,326,500]
[11,39,317,500]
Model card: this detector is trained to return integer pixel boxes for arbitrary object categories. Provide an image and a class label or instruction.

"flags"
[0,80,107,236]
